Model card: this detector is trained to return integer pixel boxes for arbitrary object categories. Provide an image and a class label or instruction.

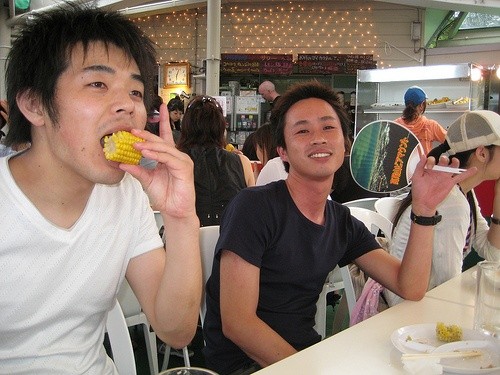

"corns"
[225,144,234,152]
[102,131,147,167]
[436,322,463,344]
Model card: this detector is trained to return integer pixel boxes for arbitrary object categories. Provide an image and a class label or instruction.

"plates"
[391,320,500,373]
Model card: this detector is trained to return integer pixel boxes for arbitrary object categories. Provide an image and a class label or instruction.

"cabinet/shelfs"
[355,62,485,146]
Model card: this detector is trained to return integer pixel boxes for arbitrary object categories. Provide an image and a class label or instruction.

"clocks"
[162,61,191,90]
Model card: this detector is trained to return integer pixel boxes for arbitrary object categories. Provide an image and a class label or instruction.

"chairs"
[100,194,408,375]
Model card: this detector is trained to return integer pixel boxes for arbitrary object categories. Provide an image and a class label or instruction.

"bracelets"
[410,208,442,227]
[490,212,500,226]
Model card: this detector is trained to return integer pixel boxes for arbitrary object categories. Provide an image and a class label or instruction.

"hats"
[441,110,500,159]
[404,87,428,107]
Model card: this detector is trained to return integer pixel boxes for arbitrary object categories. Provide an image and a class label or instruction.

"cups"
[473,260,500,338]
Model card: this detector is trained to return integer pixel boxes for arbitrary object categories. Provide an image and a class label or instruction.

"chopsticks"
[401,351,480,359]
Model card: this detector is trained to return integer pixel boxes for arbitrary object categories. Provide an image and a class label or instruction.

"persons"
[163,97,185,132]
[201,79,478,375]
[172,96,255,230]
[388,86,451,199]
[0,0,202,375]
[252,122,332,202]
[380,110,500,310]
[256,81,284,124]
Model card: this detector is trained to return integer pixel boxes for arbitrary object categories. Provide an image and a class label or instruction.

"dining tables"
[252,254,500,375]
